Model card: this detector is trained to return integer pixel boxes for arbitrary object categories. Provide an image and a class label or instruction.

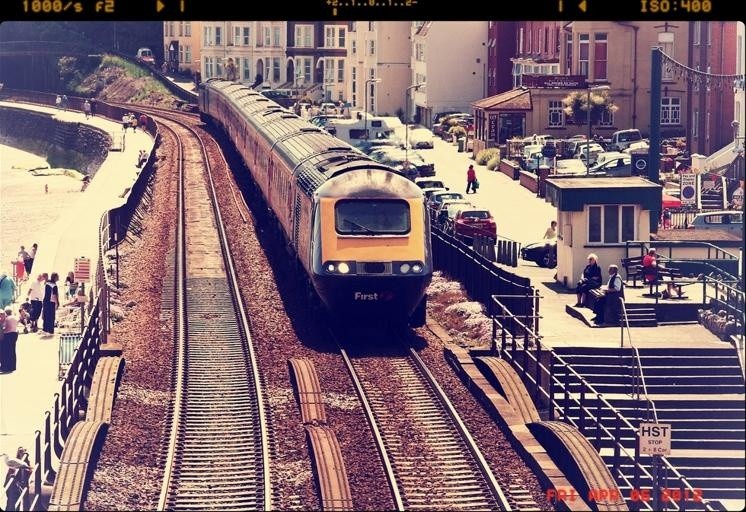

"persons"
[338,101,345,114]
[591,264,626,324]
[55,94,149,192]
[543,221,557,240]
[662,208,672,225]
[466,164,479,195]
[644,247,686,296]
[0,242,78,372]
[160,61,168,76]
[573,252,603,307]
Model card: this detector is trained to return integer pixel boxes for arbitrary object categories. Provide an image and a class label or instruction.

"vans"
[688,209,743,243]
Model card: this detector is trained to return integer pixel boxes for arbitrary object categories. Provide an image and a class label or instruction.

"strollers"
[17,298,38,334]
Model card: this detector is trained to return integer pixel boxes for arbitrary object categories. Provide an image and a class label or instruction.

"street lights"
[324,82,336,121]
[405,81,428,169]
[295,75,306,111]
[585,85,611,176]
[364,78,380,143]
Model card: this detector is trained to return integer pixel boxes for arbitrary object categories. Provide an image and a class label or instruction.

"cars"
[521,238,558,268]
[409,127,434,149]
[296,103,311,115]
[432,110,475,141]
[525,126,651,182]
[415,174,497,245]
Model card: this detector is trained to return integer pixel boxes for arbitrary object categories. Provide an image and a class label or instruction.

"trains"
[196,70,434,333]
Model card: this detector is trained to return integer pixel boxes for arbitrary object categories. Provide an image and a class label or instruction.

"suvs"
[319,102,337,115]
[137,47,156,66]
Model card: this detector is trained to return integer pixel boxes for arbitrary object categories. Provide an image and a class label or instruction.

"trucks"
[323,114,401,148]
